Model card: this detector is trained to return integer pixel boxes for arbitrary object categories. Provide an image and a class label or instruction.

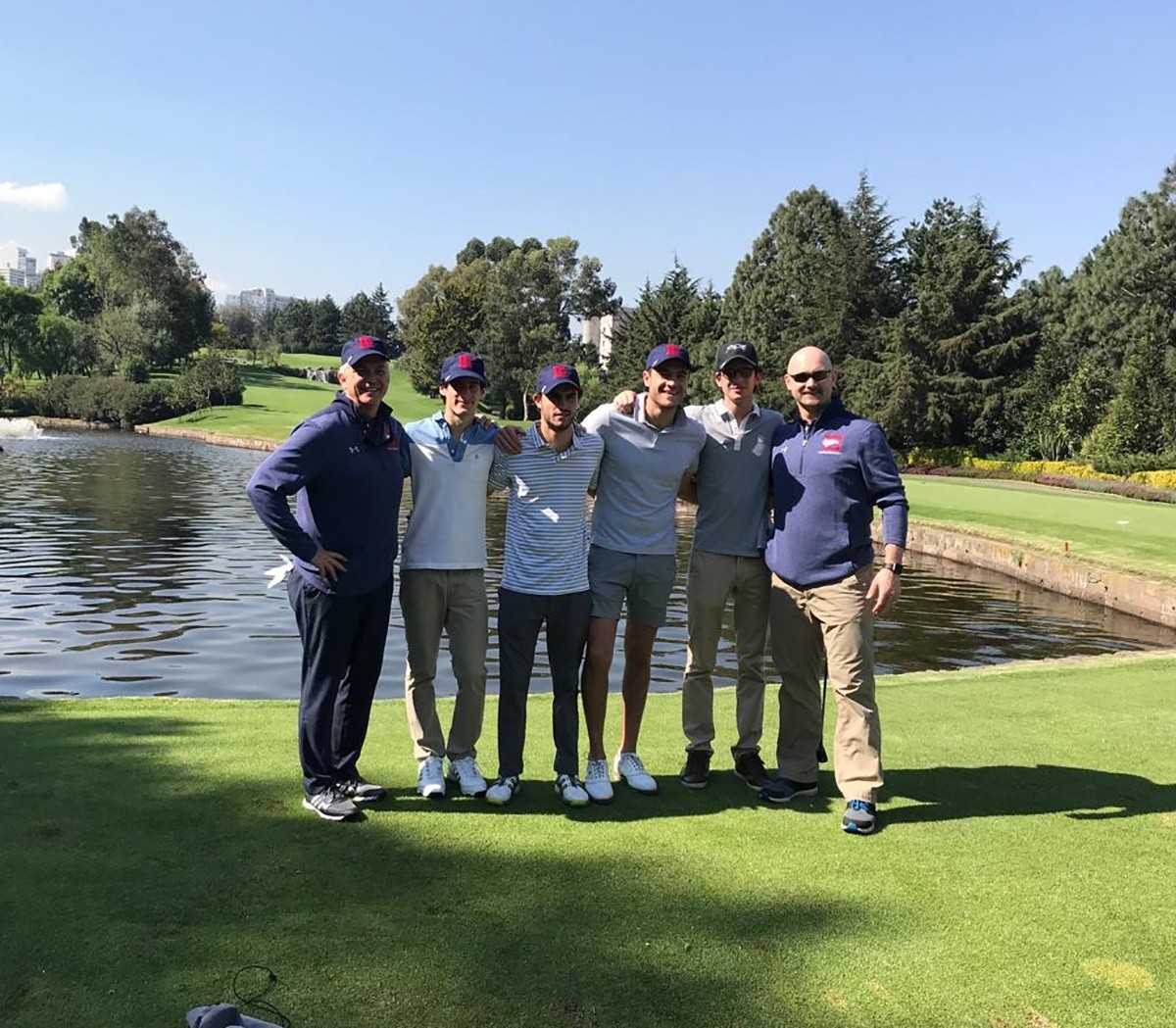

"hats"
[717,340,758,369]
[646,344,689,370]
[342,336,391,364]
[439,351,488,384]
[537,364,581,395]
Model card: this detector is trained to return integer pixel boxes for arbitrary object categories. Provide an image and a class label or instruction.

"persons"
[247,335,499,821]
[614,339,786,791]
[401,350,500,797]
[692,347,910,834]
[580,342,774,803]
[405,362,605,806]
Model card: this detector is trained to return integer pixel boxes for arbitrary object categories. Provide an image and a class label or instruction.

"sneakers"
[337,775,388,802]
[759,777,819,802]
[448,757,488,796]
[417,756,446,799]
[680,751,711,789]
[735,754,773,792]
[486,774,522,806]
[842,800,877,834]
[554,773,589,807]
[302,787,359,822]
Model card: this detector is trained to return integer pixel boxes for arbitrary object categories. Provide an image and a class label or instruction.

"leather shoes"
[615,750,659,796]
[586,759,616,805]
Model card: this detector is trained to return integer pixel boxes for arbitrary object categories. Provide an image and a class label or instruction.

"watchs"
[882,563,903,574]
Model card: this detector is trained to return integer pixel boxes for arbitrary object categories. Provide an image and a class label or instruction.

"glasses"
[723,368,754,380]
[787,370,831,383]
